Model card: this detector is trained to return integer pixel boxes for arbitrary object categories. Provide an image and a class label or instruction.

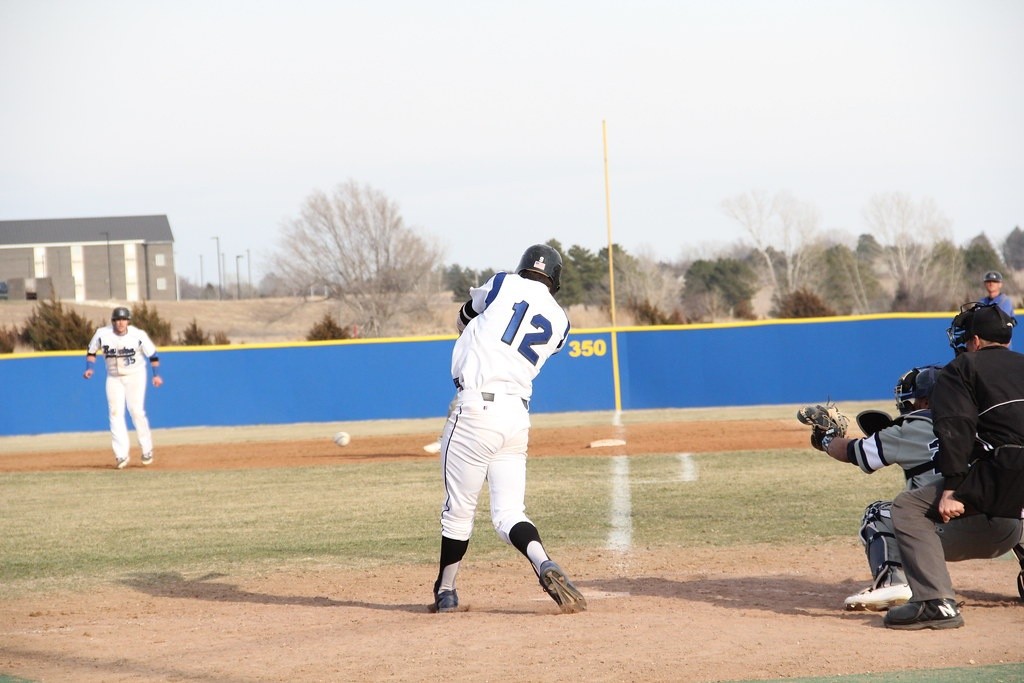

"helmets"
[111,307,131,320]
[983,271,1002,282]
[514,244,562,292]
[911,368,941,398]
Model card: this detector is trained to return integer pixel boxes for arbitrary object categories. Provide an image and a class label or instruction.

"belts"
[482,392,529,410]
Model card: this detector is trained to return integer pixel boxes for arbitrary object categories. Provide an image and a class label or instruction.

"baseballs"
[334,431,350,446]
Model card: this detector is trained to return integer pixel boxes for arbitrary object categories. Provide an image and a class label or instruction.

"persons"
[797,364,946,611]
[980,271,1015,317]
[433,244,588,614]
[882,302,1024,628]
[83,307,164,467]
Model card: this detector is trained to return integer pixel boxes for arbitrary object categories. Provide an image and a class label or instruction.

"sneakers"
[435,589,459,612]
[539,560,587,614]
[843,582,913,611]
[884,598,965,630]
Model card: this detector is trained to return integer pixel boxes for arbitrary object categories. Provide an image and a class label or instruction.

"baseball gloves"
[797,404,849,450]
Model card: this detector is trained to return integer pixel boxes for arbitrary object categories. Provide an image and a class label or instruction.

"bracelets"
[86,361,95,370]
[152,366,160,376]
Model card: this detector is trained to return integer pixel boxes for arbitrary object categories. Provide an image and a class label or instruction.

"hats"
[953,302,1013,344]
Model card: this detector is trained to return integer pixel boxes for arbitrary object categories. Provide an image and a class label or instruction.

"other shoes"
[116,456,129,468]
[141,450,152,466]
